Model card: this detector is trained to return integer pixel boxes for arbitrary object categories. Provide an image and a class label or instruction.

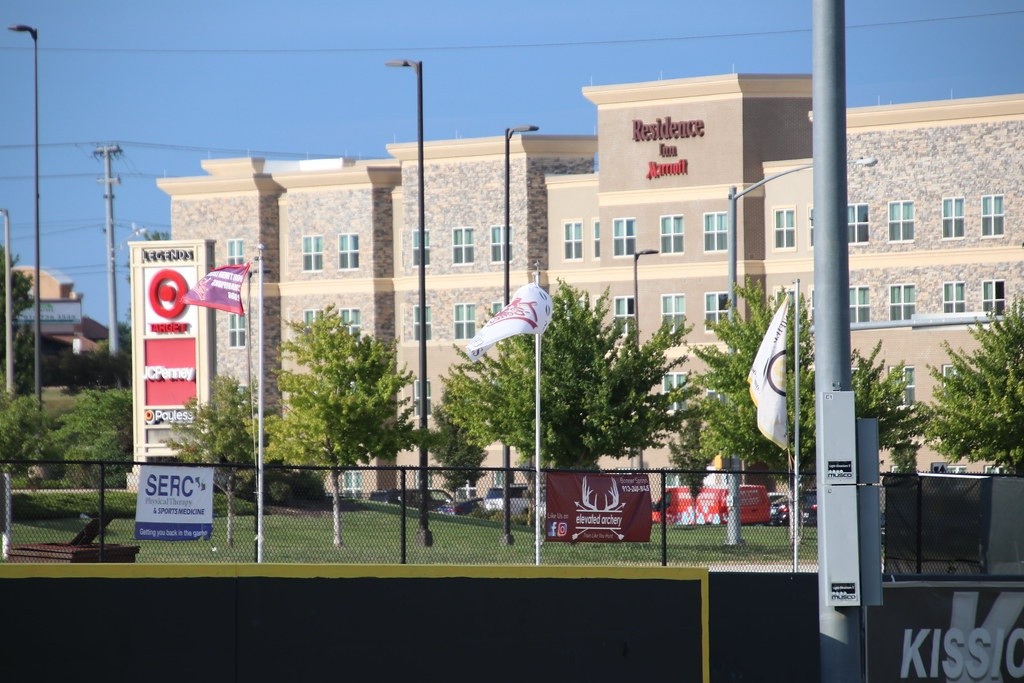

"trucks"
[651,485,771,525]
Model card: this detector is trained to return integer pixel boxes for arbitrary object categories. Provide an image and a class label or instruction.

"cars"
[528,489,547,518]
[367,489,453,511]
[484,483,546,515]
[434,497,483,517]
[765,489,818,526]
[768,492,786,502]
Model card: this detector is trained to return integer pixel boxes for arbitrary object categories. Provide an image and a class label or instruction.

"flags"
[466,283,553,361]
[179,261,249,316]
[746,291,790,450]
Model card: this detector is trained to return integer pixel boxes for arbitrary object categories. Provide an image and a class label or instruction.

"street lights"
[634,249,659,469]
[8,24,41,412]
[385,60,433,549]
[499,125,539,545]
[0,209,16,402]
[727,157,878,543]
